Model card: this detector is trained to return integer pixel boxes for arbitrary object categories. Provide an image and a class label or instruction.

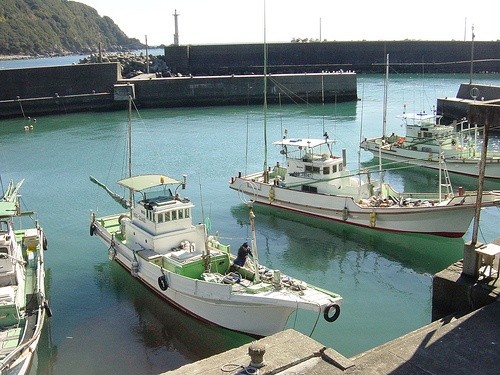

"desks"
[475,243,500,281]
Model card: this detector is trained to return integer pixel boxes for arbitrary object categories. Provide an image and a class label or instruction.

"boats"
[230,2,499,241]
[0,174,50,375]
[88,88,343,339]
[355,23,500,183]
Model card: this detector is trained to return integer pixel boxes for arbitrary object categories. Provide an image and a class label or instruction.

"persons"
[233,242,253,268]
